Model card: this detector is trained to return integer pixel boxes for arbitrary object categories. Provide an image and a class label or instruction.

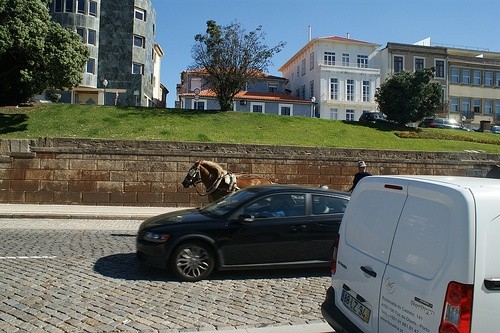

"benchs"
[316,197,346,213]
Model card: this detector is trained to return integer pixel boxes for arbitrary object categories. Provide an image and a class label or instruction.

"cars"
[490,126,500,134]
[135,184,352,282]
[471,125,484,133]
[359,111,384,124]
[418,117,475,133]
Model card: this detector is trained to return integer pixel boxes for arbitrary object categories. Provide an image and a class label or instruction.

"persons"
[345,161,373,192]
[252,197,285,217]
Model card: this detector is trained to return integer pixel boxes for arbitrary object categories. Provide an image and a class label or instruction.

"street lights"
[462,115,466,126]
[311,96,317,118]
[103,78,108,105]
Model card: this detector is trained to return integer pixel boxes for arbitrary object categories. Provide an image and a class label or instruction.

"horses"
[182,159,278,203]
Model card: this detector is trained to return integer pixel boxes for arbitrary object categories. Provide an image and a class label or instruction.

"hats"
[358,161,367,167]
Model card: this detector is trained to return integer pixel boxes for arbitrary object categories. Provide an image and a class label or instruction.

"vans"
[320,174,500,333]
